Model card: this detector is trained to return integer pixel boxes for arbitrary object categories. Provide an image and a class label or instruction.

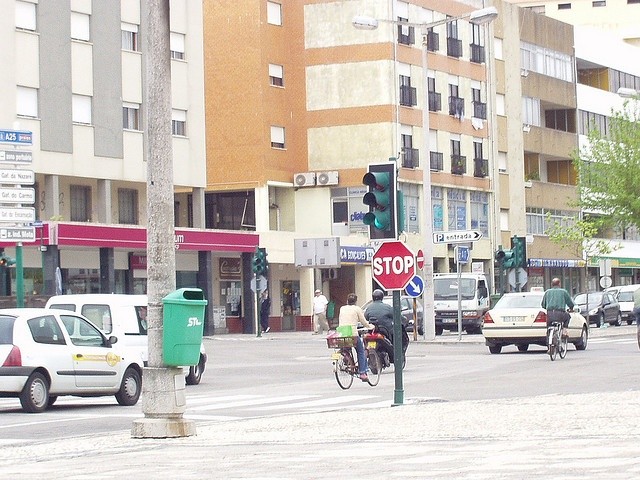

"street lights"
[351,6,498,340]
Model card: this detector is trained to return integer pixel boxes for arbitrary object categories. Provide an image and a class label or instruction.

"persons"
[541,278,573,355]
[338,294,375,382]
[260,297,272,334]
[312,289,331,336]
[365,289,407,343]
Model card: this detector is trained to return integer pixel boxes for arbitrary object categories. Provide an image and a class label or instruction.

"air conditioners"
[293,172,315,187]
[315,170,339,185]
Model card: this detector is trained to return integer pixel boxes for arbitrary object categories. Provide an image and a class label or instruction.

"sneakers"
[563,329,569,338]
[360,374,368,382]
[266,327,271,332]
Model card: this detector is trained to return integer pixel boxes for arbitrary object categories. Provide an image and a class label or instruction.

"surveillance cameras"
[37,245,47,252]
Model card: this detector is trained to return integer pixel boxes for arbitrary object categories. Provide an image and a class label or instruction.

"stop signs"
[371,241,415,291]
[417,250,424,270]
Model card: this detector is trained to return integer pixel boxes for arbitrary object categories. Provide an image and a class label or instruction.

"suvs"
[0,308,144,412]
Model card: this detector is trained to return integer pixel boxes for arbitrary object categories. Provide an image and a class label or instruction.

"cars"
[359,295,424,335]
[481,287,588,354]
[573,291,621,327]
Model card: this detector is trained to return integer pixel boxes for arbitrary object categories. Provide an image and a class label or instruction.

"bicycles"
[332,326,380,389]
[547,308,569,360]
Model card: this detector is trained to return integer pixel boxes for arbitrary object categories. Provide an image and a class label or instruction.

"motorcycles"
[362,322,412,374]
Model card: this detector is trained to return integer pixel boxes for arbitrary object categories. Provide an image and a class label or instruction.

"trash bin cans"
[161,287,208,367]
[327,301,337,320]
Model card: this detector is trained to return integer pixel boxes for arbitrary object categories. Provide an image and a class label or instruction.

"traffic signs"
[0,226,35,242]
[0,149,33,165]
[433,230,482,244]
[0,169,35,185]
[0,129,33,146]
[454,245,468,263]
[0,187,35,204]
[0,206,35,223]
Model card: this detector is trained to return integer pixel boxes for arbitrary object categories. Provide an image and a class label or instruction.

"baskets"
[327,336,358,348]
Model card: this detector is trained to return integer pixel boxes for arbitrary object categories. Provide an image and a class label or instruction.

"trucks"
[433,272,490,335]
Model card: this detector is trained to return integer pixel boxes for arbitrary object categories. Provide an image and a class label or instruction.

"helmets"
[372,289,384,301]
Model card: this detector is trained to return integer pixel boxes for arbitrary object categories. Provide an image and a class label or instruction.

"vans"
[609,284,640,325]
[44,294,207,385]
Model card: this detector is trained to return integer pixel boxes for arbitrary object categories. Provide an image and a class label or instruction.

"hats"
[315,289,320,293]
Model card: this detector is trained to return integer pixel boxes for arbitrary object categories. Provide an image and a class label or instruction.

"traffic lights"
[502,251,515,269]
[251,247,266,274]
[494,250,505,260]
[362,160,396,239]
